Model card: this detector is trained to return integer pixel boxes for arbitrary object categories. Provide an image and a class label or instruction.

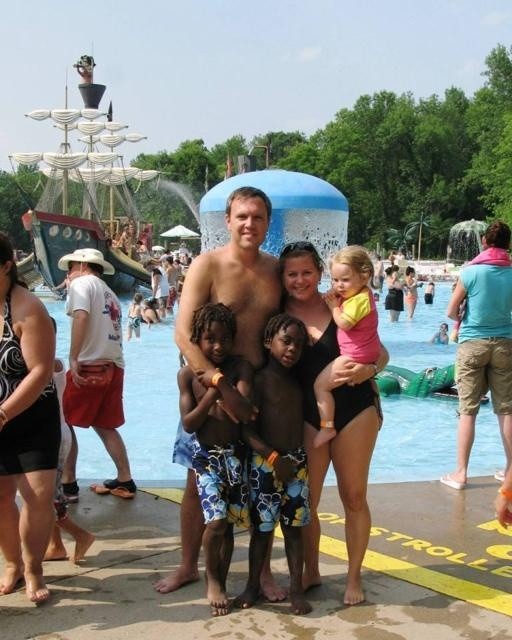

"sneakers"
[103,479,137,493]
[61,480,79,494]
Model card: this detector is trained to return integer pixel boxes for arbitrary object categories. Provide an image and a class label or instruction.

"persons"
[431,221,512,529]
[1,235,137,602]
[369,250,435,323]
[152,187,390,616]
[106,214,192,341]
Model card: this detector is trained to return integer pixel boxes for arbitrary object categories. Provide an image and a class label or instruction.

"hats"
[58,248,115,275]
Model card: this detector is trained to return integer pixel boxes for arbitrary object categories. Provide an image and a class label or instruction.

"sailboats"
[5,54,156,302]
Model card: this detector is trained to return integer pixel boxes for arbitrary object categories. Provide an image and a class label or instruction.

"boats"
[16,252,44,292]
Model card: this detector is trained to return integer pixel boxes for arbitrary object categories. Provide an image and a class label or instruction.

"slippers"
[110,486,134,499]
[440,473,466,490]
[494,468,506,481]
[90,484,109,495]
